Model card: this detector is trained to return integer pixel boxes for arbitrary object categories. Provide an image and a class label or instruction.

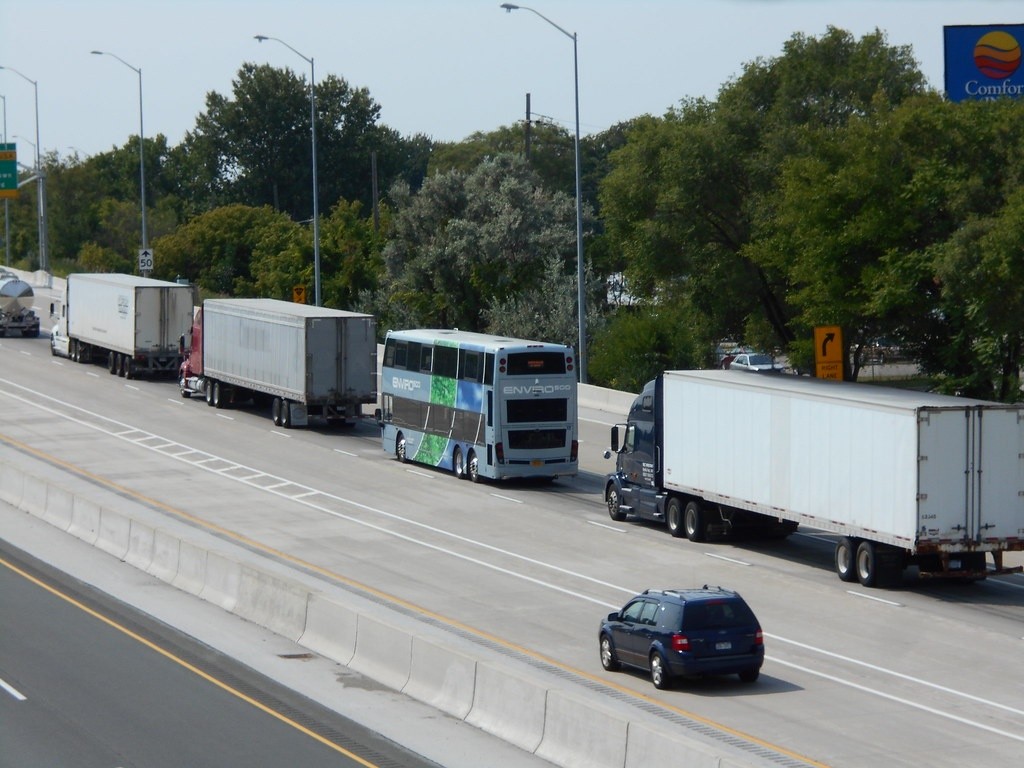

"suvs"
[597,585,767,693]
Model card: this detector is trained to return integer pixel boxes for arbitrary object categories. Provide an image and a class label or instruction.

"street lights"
[0,65,47,272]
[89,50,150,278]
[253,34,322,308]
[497,2,590,385]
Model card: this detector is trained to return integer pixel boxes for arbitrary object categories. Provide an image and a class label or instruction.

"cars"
[717,341,786,374]
[850,335,917,364]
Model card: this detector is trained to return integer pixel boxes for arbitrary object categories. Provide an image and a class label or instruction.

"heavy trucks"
[47,272,196,380]
[177,298,379,431]
[600,368,1024,590]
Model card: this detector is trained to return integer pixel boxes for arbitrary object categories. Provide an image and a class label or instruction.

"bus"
[373,327,580,486]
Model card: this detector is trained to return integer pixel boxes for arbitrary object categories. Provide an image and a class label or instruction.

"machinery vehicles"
[0,268,40,339]
